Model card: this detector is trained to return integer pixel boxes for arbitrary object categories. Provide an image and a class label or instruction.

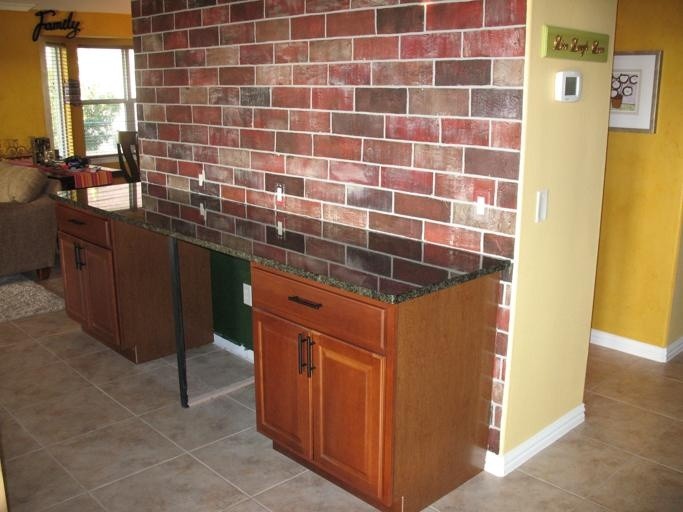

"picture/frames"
[608,51,663,133]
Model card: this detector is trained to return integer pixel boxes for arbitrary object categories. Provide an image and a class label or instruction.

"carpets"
[1,273,65,328]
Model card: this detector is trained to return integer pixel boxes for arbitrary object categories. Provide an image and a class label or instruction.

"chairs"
[116,130,139,183]
[0,161,63,282]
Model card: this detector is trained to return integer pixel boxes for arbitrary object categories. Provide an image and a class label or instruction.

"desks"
[14,159,123,189]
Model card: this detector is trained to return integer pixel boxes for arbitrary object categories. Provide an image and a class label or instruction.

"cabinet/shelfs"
[251,262,500,512]
[56,200,214,365]
